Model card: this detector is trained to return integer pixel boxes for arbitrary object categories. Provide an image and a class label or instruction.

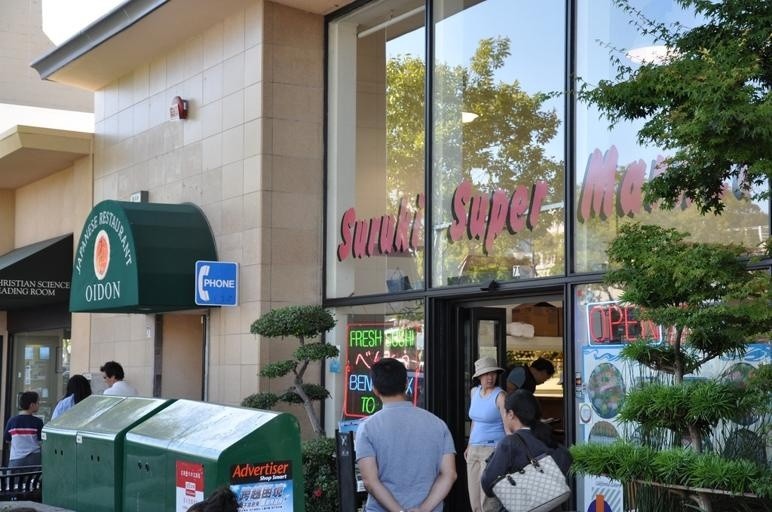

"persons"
[463,355,513,511]
[480,389,573,512]
[51,375,92,421]
[502,357,554,395]
[100,360,141,396]
[354,358,458,511]
[4,391,44,484]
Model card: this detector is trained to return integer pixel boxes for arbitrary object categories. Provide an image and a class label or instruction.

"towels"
[41,393,305,512]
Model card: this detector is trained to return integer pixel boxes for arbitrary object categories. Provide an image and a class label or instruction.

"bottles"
[472,357,504,380]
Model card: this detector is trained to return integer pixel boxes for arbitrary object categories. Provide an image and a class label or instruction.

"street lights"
[490,451,572,512]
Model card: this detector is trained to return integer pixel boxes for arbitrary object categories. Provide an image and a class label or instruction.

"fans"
[344,321,419,418]
